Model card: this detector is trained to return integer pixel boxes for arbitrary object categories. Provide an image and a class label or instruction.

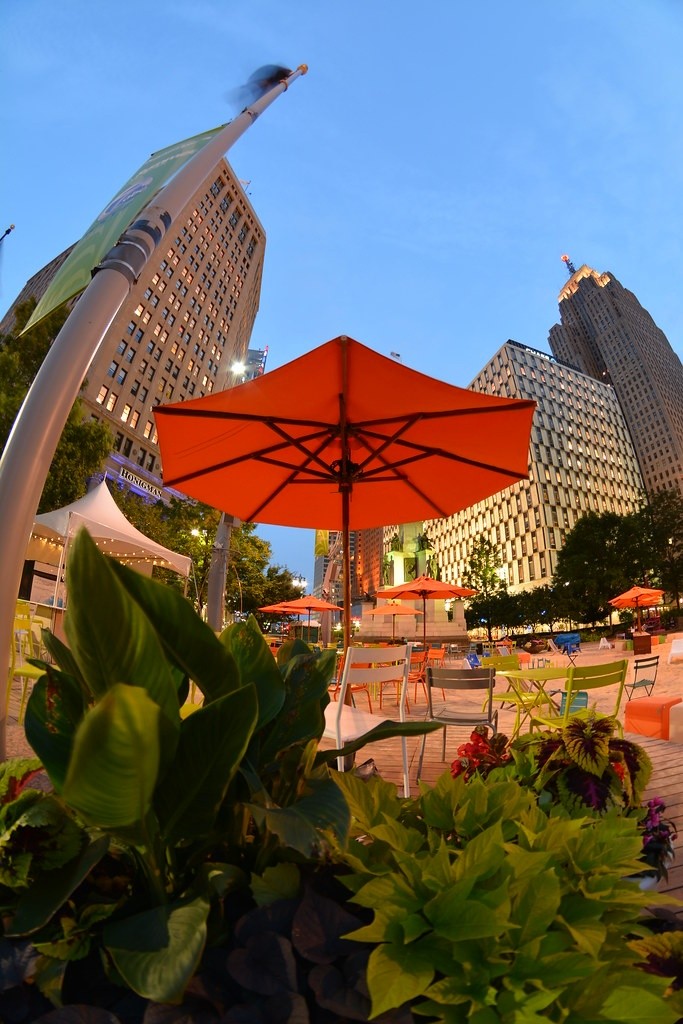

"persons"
[401,636,408,645]
[503,636,513,649]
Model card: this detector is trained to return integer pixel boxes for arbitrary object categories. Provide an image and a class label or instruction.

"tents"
[25,471,192,642]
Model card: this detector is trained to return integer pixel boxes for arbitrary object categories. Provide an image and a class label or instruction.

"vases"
[622,835,675,890]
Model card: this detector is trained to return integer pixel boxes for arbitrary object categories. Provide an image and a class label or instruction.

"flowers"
[631,795,679,885]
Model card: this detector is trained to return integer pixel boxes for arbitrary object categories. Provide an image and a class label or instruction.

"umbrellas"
[362,601,424,645]
[373,575,479,652]
[151,333,537,690]
[607,586,665,633]
[258,594,345,644]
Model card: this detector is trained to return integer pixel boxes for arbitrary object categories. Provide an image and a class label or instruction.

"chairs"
[4,600,61,727]
[267,639,660,799]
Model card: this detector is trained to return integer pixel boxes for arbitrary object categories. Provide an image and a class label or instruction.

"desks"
[495,667,572,750]
